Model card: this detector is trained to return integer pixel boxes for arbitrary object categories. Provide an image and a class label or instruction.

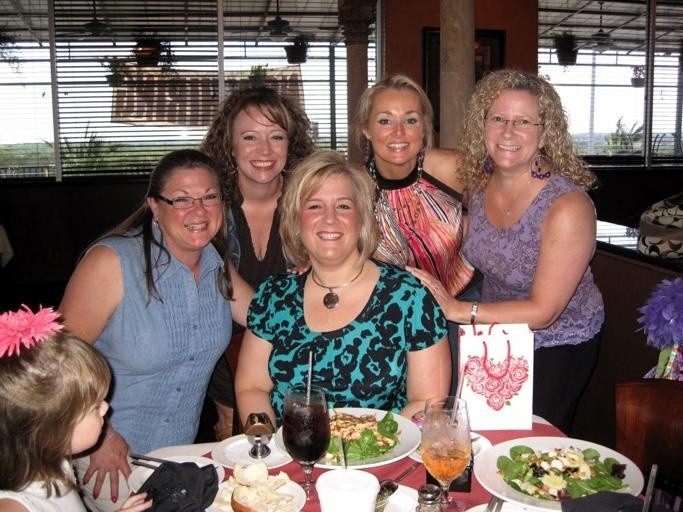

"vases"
[630,77,647,88]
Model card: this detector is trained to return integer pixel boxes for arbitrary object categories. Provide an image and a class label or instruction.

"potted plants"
[550,31,581,67]
[104,29,186,89]
[278,34,315,65]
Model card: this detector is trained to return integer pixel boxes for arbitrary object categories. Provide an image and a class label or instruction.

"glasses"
[158,192,223,210]
[485,114,543,132]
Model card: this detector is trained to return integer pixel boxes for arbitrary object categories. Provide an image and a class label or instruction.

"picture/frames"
[420,25,506,133]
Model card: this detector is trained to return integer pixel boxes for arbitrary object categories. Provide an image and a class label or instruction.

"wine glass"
[242,410,276,457]
[281,384,331,501]
[425,395,470,511]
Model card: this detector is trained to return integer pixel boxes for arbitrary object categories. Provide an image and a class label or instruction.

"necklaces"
[367,152,428,254]
[488,173,534,218]
[304,265,364,308]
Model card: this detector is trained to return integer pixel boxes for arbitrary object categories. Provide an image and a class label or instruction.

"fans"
[233,0,326,40]
[55,1,136,35]
[575,1,639,54]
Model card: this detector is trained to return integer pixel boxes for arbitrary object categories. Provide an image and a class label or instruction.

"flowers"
[634,272,682,379]
[631,63,652,78]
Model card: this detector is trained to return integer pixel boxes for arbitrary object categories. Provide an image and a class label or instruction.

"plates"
[473,436,646,511]
[127,456,225,497]
[210,434,292,470]
[202,475,306,512]
[272,406,421,467]
[313,408,491,511]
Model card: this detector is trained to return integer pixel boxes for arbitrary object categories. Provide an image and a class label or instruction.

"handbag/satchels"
[458,322,537,433]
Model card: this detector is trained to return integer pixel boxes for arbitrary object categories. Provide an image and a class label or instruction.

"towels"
[560,489,664,511]
[134,457,217,512]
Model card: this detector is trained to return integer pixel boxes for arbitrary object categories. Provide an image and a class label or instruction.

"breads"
[216,462,298,512]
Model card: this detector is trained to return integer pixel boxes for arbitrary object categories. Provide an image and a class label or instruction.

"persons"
[235,149,452,439]
[1,307,154,511]
[199,86,320,444]
[355,73,479,384]
[57,148,258,511]
[409,68,618,452]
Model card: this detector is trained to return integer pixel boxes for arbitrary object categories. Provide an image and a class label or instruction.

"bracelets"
[247,413,273,435]
[468,300,478,326]
[105,427,118,445]
